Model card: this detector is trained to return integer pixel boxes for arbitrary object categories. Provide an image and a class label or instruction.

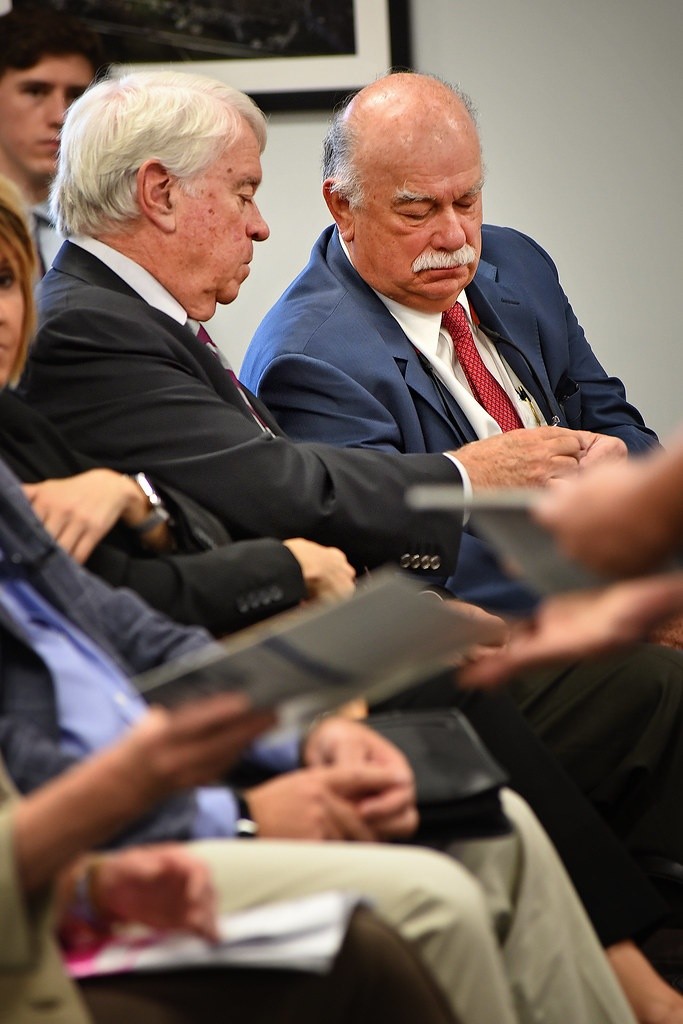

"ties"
[442,301,527,435]
[186,315,269,432]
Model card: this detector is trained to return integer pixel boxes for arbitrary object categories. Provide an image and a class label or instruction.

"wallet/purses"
[362,709,512,847]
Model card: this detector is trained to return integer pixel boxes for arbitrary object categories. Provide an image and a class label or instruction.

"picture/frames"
[13,1,408,113]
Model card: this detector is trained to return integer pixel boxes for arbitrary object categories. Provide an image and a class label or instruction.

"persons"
[0,688,278,1024]
[0,175,683,1024]
[0,12,115,279]
[13,70,582,591]
[239,70,682,612]
[0,460,643,1024]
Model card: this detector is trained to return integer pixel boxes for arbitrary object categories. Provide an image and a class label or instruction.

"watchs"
[119,470,177,538]
